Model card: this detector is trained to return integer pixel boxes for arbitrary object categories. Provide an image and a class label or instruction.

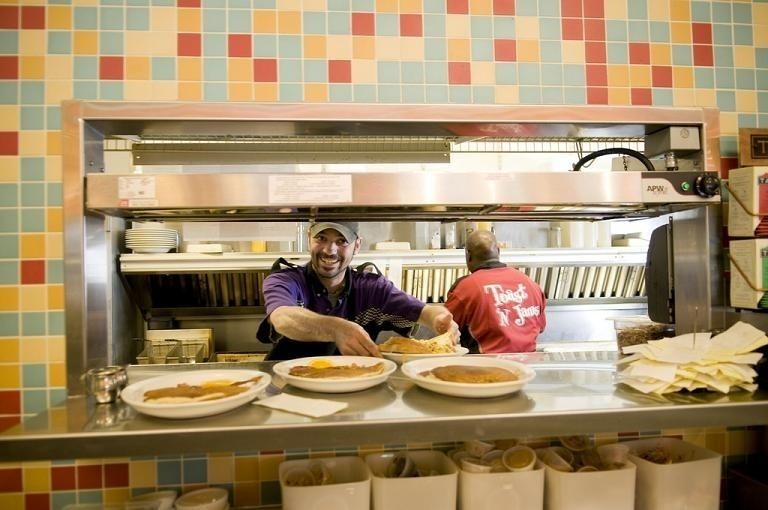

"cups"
[614,319,648,355]
[640,324,662,342]
[79,367,118,404]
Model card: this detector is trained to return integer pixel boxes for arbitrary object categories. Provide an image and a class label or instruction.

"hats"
[310,221,361,244]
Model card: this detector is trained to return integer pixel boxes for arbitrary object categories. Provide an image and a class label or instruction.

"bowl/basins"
[459,458,492,473]
[464,439,498,457]
[173,487,229,510]
[126,491,176,508]
[502,444,538,474]
[597,444,628,465]
[541,449,576,474]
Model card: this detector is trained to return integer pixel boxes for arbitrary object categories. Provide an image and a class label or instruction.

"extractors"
[117,249,648,323]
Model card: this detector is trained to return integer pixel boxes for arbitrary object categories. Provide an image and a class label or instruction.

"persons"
[444,231,546,354]
[256,222,461,362]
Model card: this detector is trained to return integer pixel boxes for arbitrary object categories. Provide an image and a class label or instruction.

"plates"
[124,228,179,255]
[119,367,273,418]
[402,354,535,398]
[272,354,397,393]
[378,346,470,367]
